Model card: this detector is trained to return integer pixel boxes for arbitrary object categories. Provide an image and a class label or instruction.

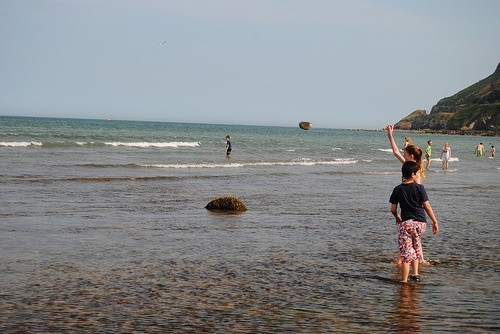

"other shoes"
[410,275,421,282]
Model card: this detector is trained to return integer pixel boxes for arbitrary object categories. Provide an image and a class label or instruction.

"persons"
[491,144,495,157]
[404,135,414,147]
[425,140,432,170]
[389,161,439,284]
[474,142,485,157]
[385,124,423,185]
[226,135,232,158]
[440,142,451,170]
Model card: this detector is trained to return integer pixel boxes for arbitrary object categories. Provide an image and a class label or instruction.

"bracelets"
[388,133,392,137]
